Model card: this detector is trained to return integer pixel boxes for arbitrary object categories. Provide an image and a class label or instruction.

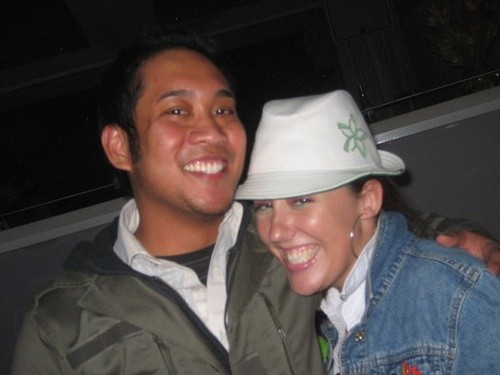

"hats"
[232,88,406,201]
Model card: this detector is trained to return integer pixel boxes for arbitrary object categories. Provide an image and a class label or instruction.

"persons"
[6,35,500,375]
[232,90,500,375]
